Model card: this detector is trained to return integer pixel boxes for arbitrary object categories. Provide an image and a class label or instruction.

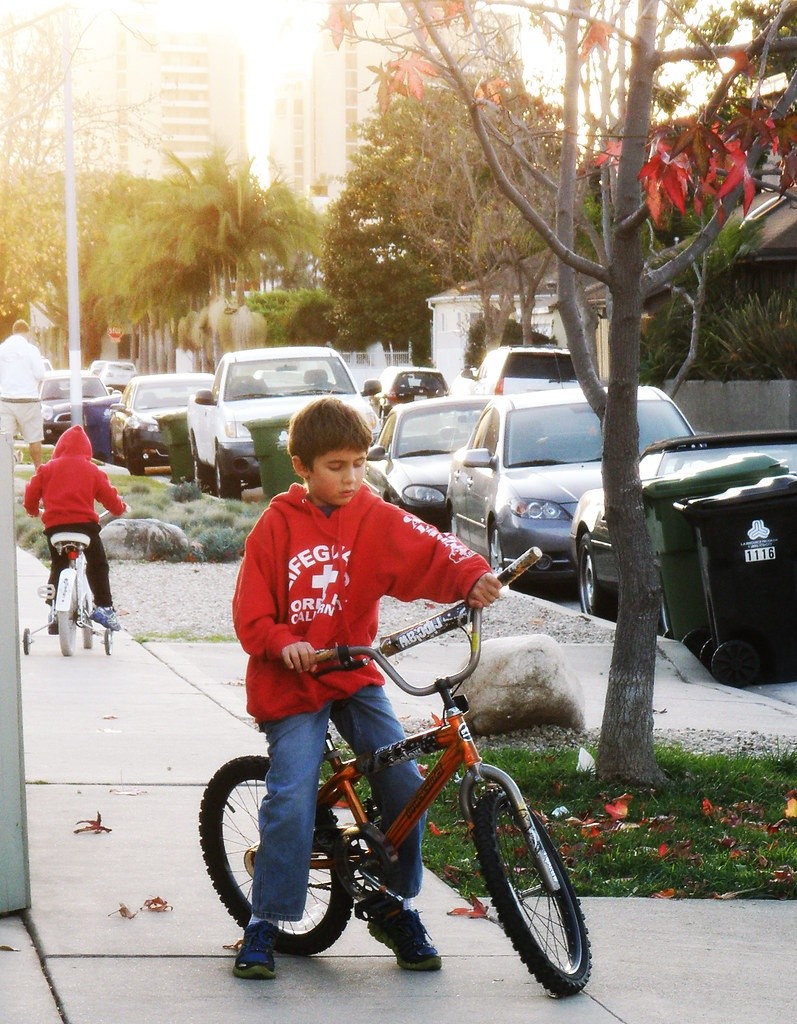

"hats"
[13,319,30,335]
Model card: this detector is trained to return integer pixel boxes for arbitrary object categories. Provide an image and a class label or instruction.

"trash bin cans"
[241,415,304,499]
[643,452,788,639]
[82,394,124,463]
[671,475,797,687]
[152,413,195,483]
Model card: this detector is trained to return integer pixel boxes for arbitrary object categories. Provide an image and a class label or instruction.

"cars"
[568,429,797,624]
[38,369,113,449]
[109,373,215,476]
[360,392,495,533]
[87,359,138,392]
[444,387,706,596]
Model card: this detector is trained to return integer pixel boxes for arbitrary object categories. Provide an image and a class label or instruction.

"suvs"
[367,365,450,420]
[460,344,580,397]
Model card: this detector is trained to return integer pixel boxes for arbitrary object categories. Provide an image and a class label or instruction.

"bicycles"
[21,504,133,657]
[196,548,596,1001]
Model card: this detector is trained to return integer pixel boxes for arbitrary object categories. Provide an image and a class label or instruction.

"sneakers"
[89,606,121,632]
[48,610,59,635]
[231,920,277,979]
[366,909,442,970]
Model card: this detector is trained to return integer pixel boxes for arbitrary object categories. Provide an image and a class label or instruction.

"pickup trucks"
[185,347,383,499]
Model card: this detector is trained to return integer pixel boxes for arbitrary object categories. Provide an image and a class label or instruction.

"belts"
[1,397,41,403]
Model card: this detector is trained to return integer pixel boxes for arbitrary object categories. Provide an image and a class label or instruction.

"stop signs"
[107,323,125,341]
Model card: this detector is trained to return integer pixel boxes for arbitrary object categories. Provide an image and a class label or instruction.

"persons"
[47,382,65,399]
[233,397,502,977]
[0,318,46,473]
[141,392,155,406]
[24,426,130,635]
